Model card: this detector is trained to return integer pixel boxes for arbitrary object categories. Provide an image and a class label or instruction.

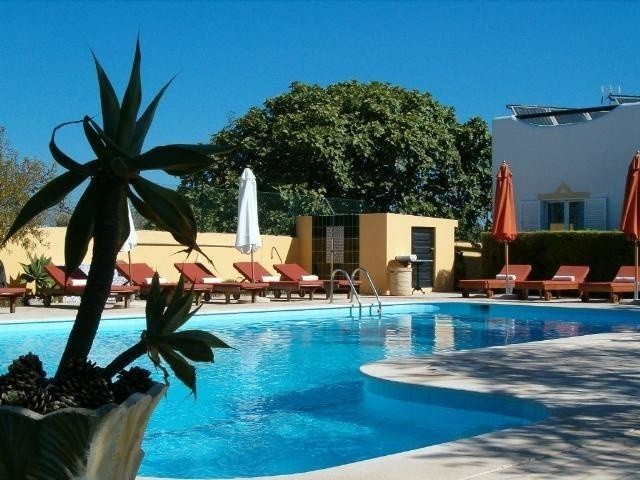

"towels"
[395,255,418,261]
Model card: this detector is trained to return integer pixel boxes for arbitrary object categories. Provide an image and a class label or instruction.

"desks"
[400,260,434,295]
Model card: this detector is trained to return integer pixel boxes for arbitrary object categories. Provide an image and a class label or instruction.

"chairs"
[459,264,640,304]
[0,260,363,313]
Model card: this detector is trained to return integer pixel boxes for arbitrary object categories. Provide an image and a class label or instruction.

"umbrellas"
[120,202,137,301]
[491,160,517,295]
[620,150,640,299]
[235,164,261,295]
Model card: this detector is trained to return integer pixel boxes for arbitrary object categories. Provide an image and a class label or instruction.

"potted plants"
[0,26,249,480]
